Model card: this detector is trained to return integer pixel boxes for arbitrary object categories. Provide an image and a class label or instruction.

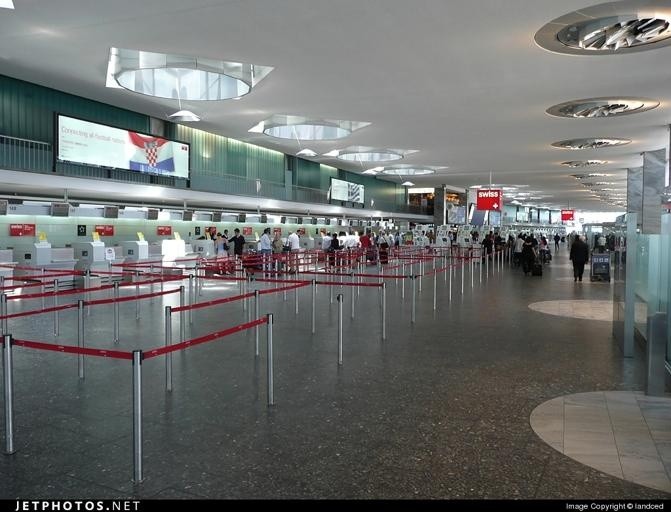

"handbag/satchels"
[381,243,389,249]
[283,246,291,250]
[223,243,230,249]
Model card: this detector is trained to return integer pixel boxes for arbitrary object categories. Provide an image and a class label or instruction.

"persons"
[205,227,607,282]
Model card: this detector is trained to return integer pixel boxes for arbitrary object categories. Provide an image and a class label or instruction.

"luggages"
[532,261,543,276]
